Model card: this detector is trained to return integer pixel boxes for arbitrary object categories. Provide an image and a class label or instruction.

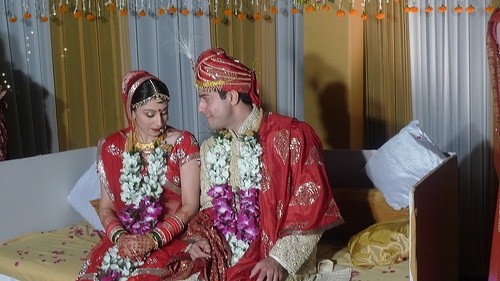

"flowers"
[0,0,500,26]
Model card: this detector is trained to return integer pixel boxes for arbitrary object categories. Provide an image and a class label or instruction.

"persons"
[0,72,13,161]
[75,69,200,281]
[173,47,345,281]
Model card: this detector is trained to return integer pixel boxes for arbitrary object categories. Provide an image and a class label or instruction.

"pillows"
[360,119,450,210]
[343,207,410,269]
[361,188,411,224]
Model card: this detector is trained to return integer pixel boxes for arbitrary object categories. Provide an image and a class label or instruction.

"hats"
[193,47,262,106]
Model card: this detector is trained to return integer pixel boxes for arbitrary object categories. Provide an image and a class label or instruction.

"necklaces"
[131,127,168,152]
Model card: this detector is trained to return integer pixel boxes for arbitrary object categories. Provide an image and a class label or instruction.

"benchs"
[0,136,464,281]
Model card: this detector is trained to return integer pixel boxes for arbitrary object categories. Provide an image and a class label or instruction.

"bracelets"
[146,215,184,250]
[105,217,128,244]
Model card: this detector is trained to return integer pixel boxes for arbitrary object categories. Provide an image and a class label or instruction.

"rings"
[131,248,137,253]
